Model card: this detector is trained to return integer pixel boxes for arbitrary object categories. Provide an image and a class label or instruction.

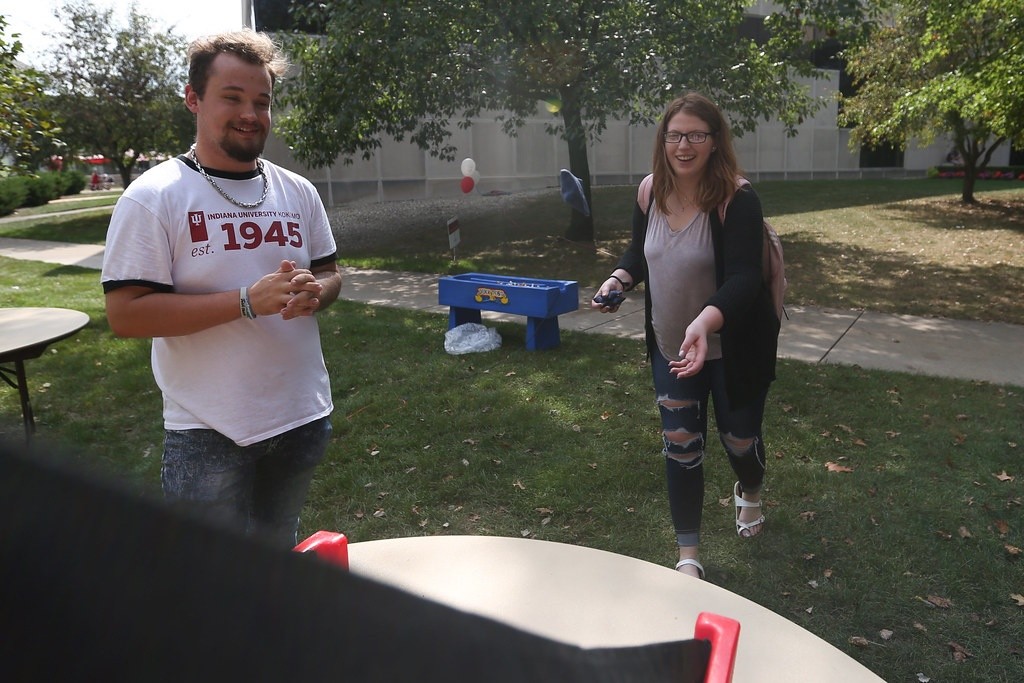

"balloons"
[461,177,474,194]
[471,170,480,184]
[461,158,475,176]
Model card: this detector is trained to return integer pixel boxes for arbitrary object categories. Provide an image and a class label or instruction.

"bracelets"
[609,276,626,290]
[240,287,256,320]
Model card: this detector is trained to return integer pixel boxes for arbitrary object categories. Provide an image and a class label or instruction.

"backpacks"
[638,172,787,322]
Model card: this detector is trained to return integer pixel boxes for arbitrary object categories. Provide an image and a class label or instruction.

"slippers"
[675,558,705,579]
[733,480,766,541]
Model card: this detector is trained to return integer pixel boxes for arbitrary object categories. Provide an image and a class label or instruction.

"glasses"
[661,131,712,144]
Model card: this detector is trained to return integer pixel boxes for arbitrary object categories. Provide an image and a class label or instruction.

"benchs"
[88,183,115,191]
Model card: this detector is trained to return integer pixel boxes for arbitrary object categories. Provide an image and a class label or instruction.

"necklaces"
[189,144,269,208]
[676,192,693,212]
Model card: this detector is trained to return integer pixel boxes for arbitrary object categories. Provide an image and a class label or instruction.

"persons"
[590,93,778,580]
[92,169,102,189]
[102,30,342,556]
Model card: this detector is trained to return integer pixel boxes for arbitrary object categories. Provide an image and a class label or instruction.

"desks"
[347,535,887,683]
[0,307,90,448]
